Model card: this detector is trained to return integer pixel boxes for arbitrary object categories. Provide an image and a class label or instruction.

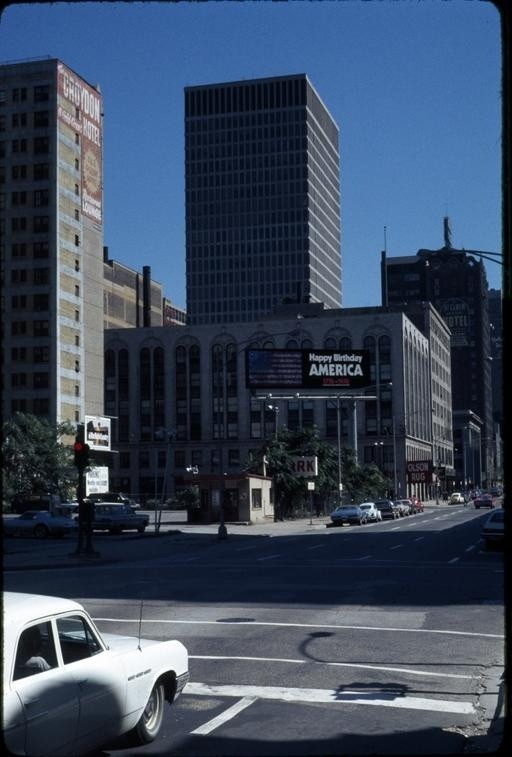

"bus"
[11,494,60,513]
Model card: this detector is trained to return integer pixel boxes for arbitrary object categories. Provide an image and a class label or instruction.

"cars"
[3,590,190,755]
[329,497,424,524]
[447,485,503,508]
[60,498,89,513]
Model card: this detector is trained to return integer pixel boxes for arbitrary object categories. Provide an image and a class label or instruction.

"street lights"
[392,408,436,499]
[352,382,393,472]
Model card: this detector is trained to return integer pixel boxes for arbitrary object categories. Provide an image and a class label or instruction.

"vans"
[88,493,141,510]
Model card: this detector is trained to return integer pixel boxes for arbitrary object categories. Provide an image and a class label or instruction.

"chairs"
[15,636,50,678]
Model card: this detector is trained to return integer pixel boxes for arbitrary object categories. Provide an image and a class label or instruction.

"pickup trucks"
[5,511,79,540]
[75,502,149,533]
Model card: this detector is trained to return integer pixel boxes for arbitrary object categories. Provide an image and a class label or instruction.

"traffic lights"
[74,435,85,467]
